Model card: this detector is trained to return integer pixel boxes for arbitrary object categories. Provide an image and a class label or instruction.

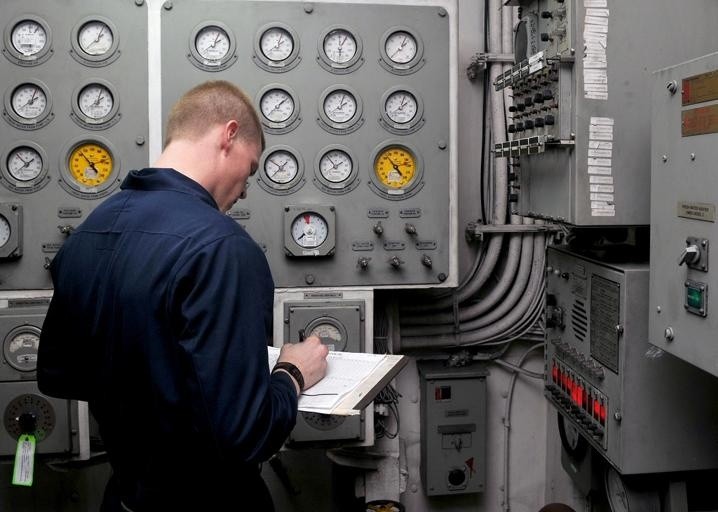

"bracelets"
[271,362,304,391]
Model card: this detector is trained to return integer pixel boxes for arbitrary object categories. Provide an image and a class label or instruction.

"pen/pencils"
[298,329,305,342]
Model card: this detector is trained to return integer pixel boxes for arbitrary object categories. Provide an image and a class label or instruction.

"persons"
[37,80,328,512]
